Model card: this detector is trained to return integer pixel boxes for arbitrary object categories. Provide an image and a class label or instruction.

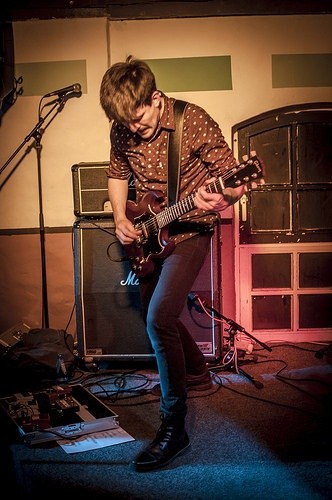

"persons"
[101,60,245,470]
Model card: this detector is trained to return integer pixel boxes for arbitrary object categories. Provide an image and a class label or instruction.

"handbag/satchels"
[2,329,78,387]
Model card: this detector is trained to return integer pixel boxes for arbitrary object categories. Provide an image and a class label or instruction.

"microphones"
[45,82,82,98]
[187,292,199,301]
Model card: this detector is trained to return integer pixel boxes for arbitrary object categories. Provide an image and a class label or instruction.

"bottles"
[55,352,69,385]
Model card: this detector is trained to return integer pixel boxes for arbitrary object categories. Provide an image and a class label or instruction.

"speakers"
[73,219,224,365]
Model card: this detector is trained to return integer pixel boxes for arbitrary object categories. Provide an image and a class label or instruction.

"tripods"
[199,299,272,389]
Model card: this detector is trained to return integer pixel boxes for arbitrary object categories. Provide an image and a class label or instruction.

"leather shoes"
[132,417,190,472]
[154,368,212,394]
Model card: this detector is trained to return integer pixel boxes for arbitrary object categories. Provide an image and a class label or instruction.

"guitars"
[124,150,266,281]
